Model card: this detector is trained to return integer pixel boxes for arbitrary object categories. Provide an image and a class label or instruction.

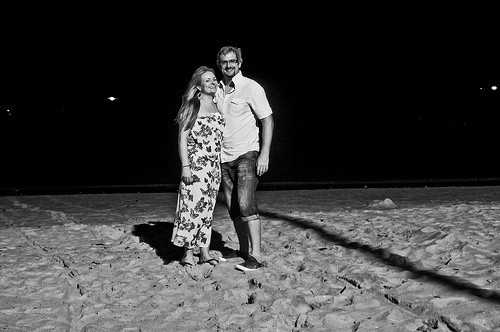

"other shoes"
[235,256,265,272]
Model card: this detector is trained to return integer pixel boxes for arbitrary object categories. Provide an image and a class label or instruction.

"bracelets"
[181,164,190,167]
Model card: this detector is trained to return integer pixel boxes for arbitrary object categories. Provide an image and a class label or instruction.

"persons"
[211,47,274,272]
[171,66,226,265]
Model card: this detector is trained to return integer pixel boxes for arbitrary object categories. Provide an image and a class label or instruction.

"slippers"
[182,256,219,267]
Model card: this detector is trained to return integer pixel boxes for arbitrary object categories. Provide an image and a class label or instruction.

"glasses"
[220,60,238,63]
